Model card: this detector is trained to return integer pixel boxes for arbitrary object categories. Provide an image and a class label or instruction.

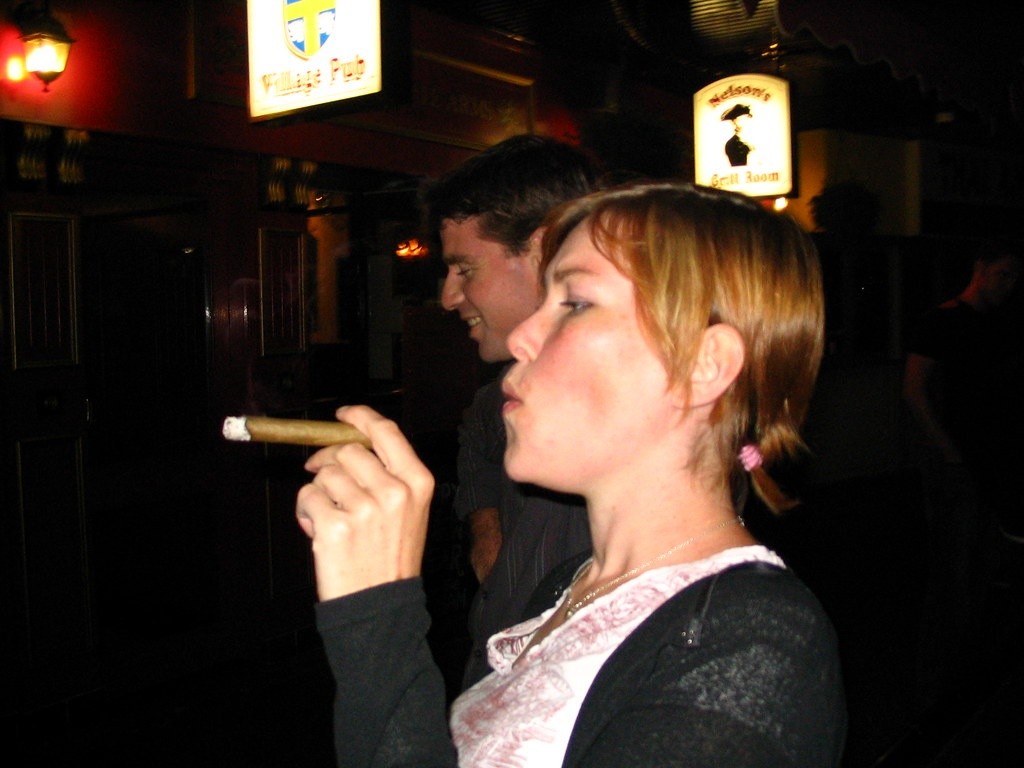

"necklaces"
[563,516,745,619]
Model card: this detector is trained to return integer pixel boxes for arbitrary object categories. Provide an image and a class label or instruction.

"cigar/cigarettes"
[221,415,373,450]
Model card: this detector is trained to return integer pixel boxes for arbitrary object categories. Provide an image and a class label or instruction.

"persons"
[900,238,1023,699]
[295,180,853,768]
[424,137,610,683]
